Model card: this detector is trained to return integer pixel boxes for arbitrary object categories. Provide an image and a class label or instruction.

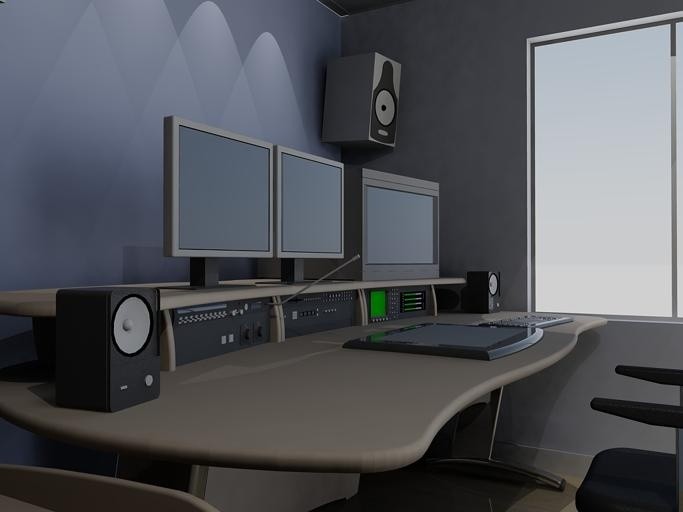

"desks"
[0,273,611,512]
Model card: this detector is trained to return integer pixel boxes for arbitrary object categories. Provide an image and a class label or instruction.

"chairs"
[573,362,681,510]
